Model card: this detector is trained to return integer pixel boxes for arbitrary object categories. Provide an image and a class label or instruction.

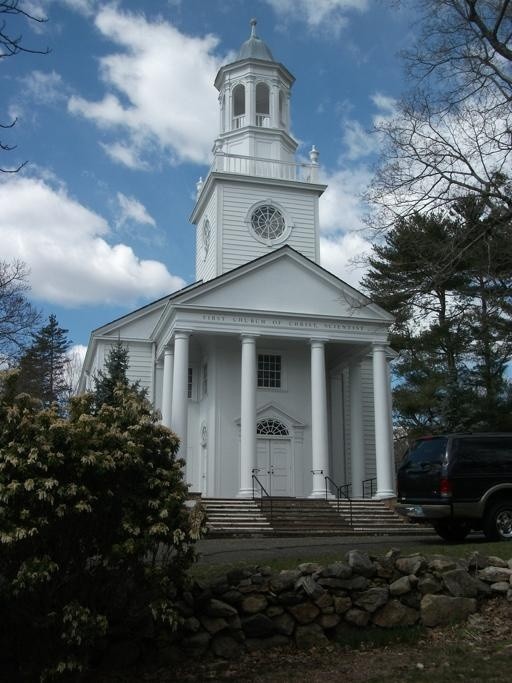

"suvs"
[391,432,512,542]
